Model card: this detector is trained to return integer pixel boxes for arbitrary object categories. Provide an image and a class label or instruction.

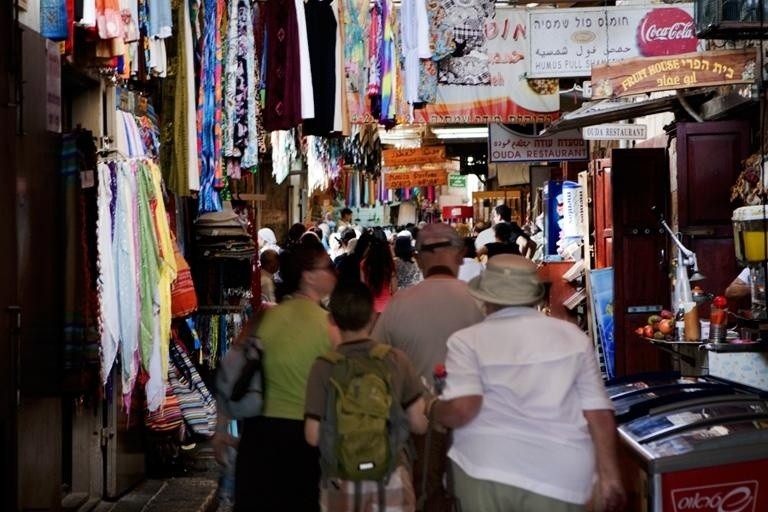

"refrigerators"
[604,372,768,511]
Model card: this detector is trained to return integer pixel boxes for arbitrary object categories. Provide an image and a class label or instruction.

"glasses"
[314,263,337,273]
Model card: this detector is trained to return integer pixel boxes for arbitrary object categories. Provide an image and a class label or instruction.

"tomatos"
[713,296,727,309]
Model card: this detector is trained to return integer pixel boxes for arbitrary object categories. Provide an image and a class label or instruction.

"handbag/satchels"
[213,340,265,419]
[157,239,198,319]
[140,347,218,437]
[164,441,198,477]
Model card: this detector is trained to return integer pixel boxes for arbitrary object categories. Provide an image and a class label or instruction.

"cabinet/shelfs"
[603,374,768,510]
[589,148,671,376]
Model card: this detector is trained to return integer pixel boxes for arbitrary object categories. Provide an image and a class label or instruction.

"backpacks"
[318,343,411,481]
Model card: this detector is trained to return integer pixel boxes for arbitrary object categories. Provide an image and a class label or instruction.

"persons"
[211,205,628,512]
[725,267,765,298]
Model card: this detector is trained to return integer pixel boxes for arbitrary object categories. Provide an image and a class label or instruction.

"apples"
[659,319,672,333]
[636,325,654,337]
[661,310,672,319]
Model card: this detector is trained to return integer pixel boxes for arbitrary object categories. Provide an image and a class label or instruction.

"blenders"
[732,205,768,344]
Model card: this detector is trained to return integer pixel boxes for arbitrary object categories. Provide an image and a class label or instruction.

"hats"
[416,223,465,251]
[467,253,546,306]
